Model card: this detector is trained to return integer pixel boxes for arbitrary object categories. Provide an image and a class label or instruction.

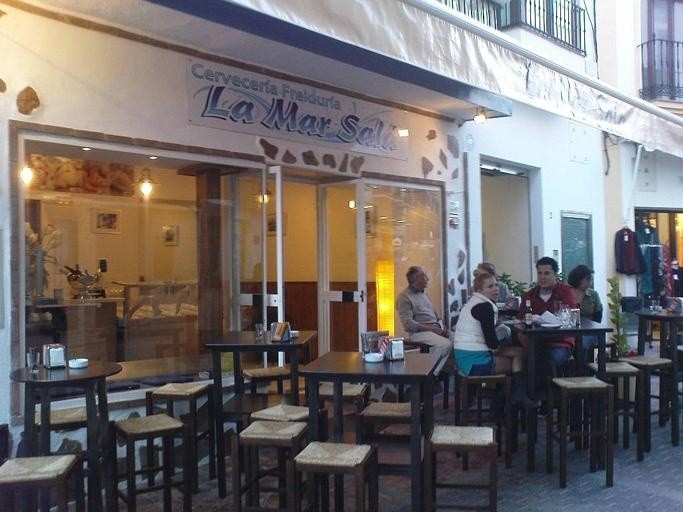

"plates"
[541,324,560,328]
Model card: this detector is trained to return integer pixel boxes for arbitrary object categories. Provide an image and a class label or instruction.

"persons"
[568,265,602,350]
[397,267,455,383]
[469,263,510,306]
[454,270,541,416]
[517,257,576,418]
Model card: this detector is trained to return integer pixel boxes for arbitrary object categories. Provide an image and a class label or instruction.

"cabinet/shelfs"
[108,279,198,363]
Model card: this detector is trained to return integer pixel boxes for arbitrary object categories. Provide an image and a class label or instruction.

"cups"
[253,322,263,341]
[366,338,378,353]
[26,352,39,372]
[651,299,660,313]
[554,300,576,328]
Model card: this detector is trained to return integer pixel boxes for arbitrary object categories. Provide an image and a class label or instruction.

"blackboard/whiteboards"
[622,297,645,334]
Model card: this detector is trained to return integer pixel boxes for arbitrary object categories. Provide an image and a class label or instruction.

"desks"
[7,360,122,511]
[23,296,258,361]
[299,349,442,512]
[501,315,615,473]
[204,330,319,499]
[633,308,683,423]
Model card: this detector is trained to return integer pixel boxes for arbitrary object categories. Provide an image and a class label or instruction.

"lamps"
[256,186,272,205]
[136,167,155,200]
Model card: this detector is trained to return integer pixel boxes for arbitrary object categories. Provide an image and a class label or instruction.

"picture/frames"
[264,212,286,236]
[90,207,122,236]
[160,223,179,247]
[354,205,376,239]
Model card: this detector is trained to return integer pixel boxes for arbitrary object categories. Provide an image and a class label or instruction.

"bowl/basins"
[496,303,505,307]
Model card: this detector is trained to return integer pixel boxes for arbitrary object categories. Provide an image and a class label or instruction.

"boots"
[489,383,517,418]
[511,370,542,411]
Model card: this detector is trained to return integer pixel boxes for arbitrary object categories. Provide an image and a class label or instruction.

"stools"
[243,402,309,512]
[357,400,423,512]
[619,353,679,448]
[454,373,506,472]
[317,381,369,443]
[35,405,100,512]
[240,366,293,412]
[544,375,614,489]
[590,360,644,472]
[295,440,379,512]
[145,378,217,493]
[239,418,308,512]
[104,409,194,512]
[2,456,78,512]
[428,422,498,512]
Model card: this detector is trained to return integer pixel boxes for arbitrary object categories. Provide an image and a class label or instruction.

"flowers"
[23,220,65,296]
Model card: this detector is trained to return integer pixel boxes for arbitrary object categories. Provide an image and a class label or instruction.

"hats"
[568,264,595,287]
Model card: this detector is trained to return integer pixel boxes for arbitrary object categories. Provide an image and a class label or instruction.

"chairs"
[360,330,391,352]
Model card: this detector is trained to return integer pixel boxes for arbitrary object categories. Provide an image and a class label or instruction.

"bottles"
[58,264,82,281]
[524,297,532,328]
